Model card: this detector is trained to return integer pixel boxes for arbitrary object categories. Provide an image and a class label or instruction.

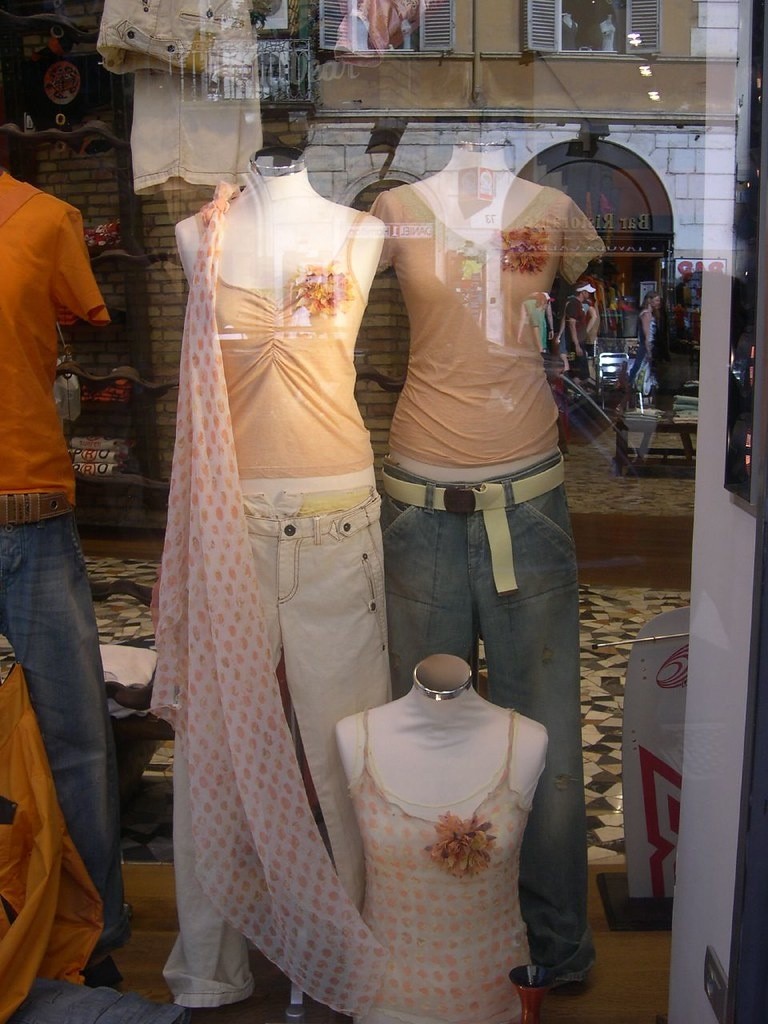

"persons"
[369,143,607,997]
[334,653,550,1024]
[149,144,393,1016]
[0,168,127,993]
[517,274,663,433]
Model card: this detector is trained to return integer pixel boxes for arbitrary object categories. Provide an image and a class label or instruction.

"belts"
[0,492,72,524]
[380,446,566,596]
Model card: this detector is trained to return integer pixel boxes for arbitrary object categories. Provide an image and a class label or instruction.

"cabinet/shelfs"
[1,10,181,535]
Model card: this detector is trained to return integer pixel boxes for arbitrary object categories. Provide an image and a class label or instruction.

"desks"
[613,412,697,478]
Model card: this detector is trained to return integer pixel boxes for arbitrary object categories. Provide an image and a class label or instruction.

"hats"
[575,284,596,293]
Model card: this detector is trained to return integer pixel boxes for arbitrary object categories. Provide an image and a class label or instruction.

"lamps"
[366,119,409,180]
[288,111,313,146]
[577,122,610,153]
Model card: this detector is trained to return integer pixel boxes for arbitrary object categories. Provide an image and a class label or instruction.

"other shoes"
[79,954,123,989]
